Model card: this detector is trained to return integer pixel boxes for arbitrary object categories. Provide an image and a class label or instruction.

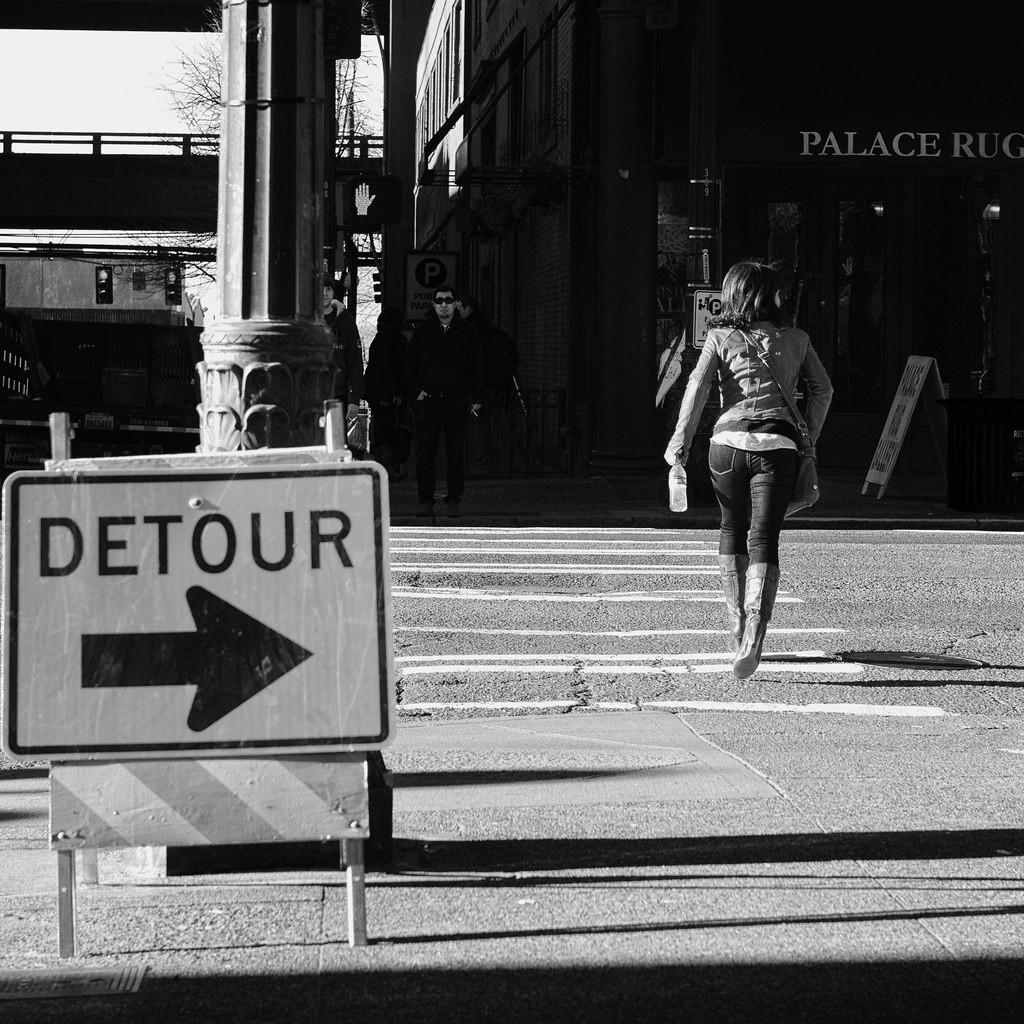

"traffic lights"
[96,265,114,304]
[339,174,407,229]
[164,267,182,307]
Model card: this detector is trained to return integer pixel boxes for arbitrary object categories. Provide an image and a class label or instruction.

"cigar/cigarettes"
[473,412,478,417]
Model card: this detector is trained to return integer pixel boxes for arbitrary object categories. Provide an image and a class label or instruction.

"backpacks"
[483,328,519,392]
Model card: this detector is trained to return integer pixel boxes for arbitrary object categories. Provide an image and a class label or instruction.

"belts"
[429,390,462,401]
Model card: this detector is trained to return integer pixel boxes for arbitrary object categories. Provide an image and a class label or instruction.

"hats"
[323,274,336,290]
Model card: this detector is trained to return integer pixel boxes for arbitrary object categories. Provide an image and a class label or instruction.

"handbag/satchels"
[784,428,820,517]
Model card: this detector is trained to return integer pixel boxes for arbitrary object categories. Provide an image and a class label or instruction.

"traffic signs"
[1,457,397,763]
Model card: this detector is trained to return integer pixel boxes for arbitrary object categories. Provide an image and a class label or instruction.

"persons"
[364,305,414,476]
[457,296,518,480]
[664,262,834,679]
[404,286,485,517]
[322,276,364,405]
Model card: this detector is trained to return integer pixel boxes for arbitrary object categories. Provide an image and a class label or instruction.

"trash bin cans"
[937,397,1024,510]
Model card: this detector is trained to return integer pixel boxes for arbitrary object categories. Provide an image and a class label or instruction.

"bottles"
[670,461,687,512]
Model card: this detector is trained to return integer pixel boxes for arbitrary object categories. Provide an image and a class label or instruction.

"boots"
[732,562,780,678]
[718,555,751,656]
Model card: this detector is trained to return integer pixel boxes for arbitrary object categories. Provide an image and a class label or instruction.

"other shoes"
[415,498,436,517]
[391,472,408,484]
[445,495,463,516]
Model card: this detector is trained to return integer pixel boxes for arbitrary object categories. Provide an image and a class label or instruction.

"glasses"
[435,297,455,304]
